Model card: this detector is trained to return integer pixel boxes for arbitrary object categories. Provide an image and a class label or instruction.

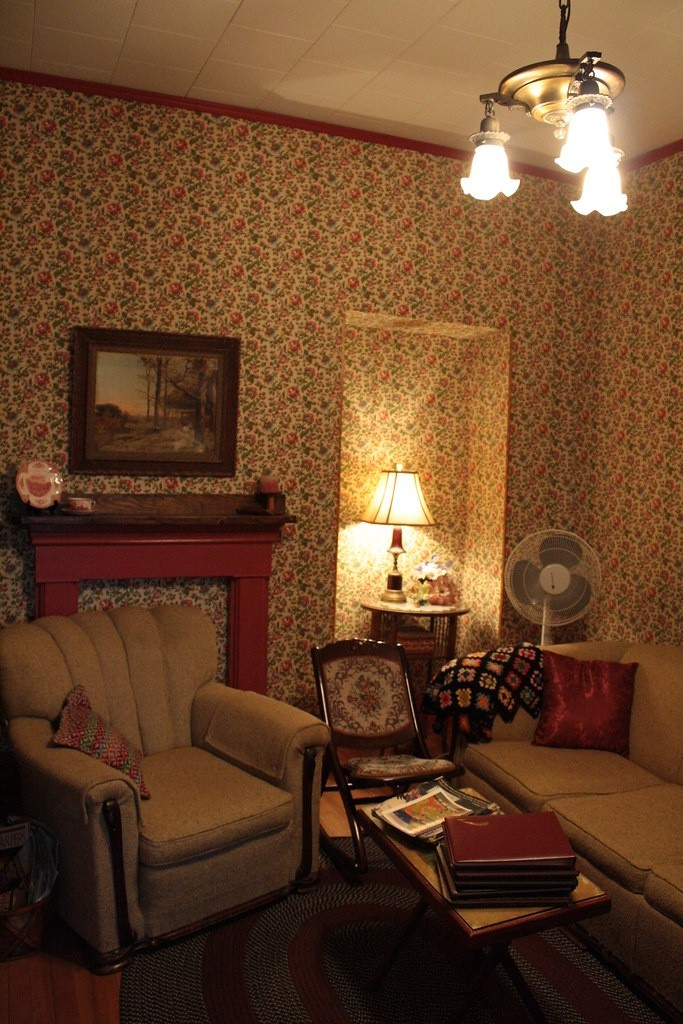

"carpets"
[120,830,672,1023]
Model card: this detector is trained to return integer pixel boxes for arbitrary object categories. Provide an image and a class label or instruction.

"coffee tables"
[358,785,612,1024]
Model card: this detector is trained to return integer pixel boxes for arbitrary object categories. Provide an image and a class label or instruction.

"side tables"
[362,599,471,680]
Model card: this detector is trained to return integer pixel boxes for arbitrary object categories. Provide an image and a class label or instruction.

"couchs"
[0,606,331,972]
[421,636,682,1021]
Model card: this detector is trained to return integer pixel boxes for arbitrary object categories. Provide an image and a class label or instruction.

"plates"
[16,459,63,508]
[62,508,96,515]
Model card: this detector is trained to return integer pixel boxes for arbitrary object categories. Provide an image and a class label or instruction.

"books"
[371,776,580,907]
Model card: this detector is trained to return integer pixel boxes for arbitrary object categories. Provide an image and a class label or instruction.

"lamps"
[456,1,630,216]
[355,469,437,605]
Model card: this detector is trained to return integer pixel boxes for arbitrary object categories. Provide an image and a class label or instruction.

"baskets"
[0,890,55,962]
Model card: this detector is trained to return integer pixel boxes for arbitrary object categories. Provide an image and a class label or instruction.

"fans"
[503,529,602,644]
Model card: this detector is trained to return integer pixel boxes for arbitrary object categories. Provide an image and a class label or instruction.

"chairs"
[304,636,469,872]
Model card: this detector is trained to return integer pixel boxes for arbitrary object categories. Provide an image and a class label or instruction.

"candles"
[260,476,279,493]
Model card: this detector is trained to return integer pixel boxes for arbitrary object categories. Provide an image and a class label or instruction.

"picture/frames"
[68,322,240,477]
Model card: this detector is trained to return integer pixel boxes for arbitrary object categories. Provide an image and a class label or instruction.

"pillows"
[53,683,150,799]
[530,648,639,760]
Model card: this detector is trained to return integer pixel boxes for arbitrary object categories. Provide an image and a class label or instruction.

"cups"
[66,498,96,510]
